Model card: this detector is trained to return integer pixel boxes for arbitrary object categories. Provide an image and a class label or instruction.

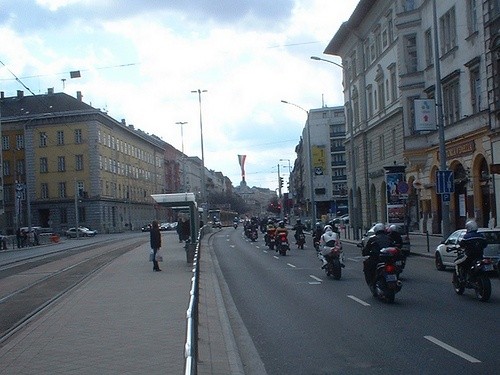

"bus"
[209,209,239,227]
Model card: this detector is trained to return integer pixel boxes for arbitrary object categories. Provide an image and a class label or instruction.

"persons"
[363,223,403,285]
[16,228,41,248]
[266,215,287,227]
[275,220,290,252]
[150,221,162,272]
[177,217,190,248]
[291,219,306,245]
[234,217,239,225]
[264,219,277,246]
[460,220,488,281]
[312,222,324,252]
[244,220,258,238]
[317,221,345,268]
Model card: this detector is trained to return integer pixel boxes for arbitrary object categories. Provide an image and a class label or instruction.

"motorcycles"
[272,232,289,257]
[446,243,494,302]
[315,242,345,280]
[357,234,404,304]
[262,227,276,250]
[311,234,320,254]
[292,229,306,250]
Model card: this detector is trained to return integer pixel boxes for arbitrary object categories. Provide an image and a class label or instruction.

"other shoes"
[340,261,345,268]
[321,261,328,268]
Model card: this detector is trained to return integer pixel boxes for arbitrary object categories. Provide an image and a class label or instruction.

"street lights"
[279,158,290,172]
[191,89,208,226]
[310,56,360,241]
[176,121,188,192]
[281,100,316,229]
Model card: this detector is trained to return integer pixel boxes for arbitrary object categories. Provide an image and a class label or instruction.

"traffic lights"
[280,177,284,188]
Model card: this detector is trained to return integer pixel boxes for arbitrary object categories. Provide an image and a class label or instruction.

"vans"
[19,226,55,236]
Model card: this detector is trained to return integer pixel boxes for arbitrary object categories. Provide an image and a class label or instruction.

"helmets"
[296,219,301,223]
[315,222,320,226]
[388,224,400,233]
[268,219,272,223]
[324,225,333,232]
[465,220,478,233]
[373,223,387,235]
[278,221,285,226]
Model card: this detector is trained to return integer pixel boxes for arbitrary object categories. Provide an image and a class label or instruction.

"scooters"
[233,220,238,230]
[240,219,280,242]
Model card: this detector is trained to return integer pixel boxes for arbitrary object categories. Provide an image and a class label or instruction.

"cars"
[434,228,500,277]
[303,213,349,230]
[360,224,411,256]
[66,225,98,239]
[141,223,153,232]
[158,221,178,231]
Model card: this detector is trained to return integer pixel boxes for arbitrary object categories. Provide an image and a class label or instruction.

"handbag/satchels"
[149,249,154,262]
[155,248,164,262]
